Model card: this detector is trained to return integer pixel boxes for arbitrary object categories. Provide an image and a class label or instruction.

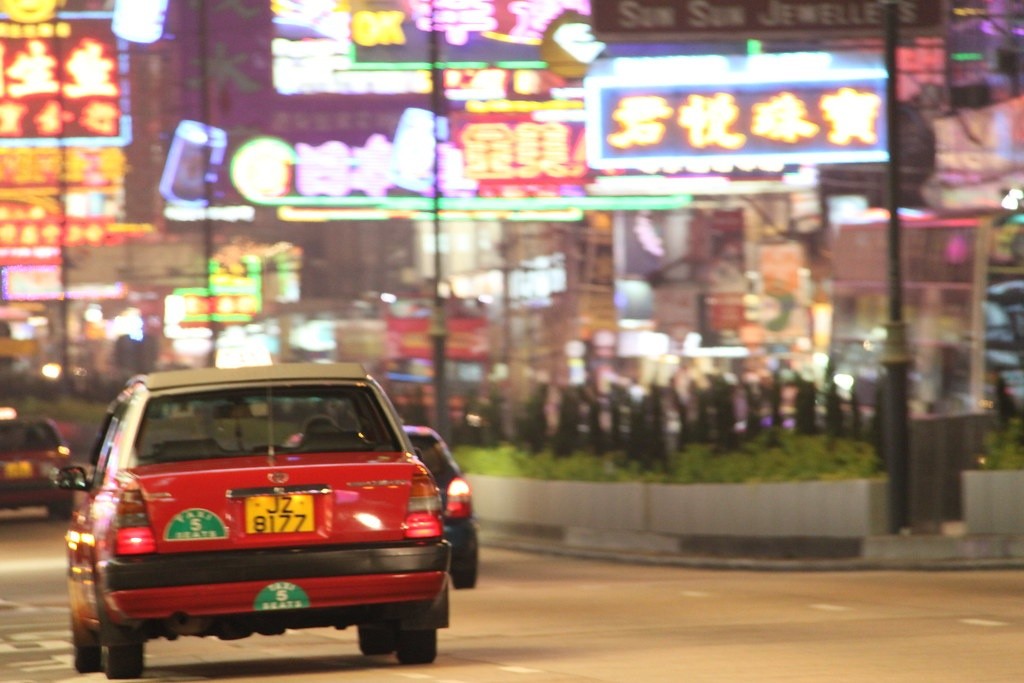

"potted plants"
[960,417,1024,534]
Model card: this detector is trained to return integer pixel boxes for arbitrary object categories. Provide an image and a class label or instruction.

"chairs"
[303,431,371,450]
[153,438,223,461]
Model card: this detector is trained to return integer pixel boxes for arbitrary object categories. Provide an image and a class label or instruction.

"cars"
[281,424,479,592]
[0,413,77,521]
[52,361,453,678]
